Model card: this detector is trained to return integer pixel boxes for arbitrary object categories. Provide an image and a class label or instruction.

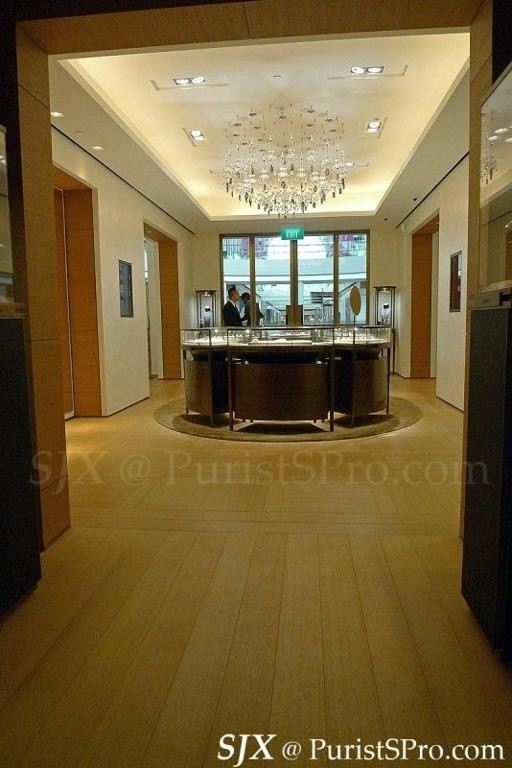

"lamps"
[224,102,345,219]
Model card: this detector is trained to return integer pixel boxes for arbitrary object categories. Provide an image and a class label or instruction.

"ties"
[235,305,237,310]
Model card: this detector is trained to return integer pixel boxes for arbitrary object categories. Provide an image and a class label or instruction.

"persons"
[223,288,242,326]
[241,293,264,326]
